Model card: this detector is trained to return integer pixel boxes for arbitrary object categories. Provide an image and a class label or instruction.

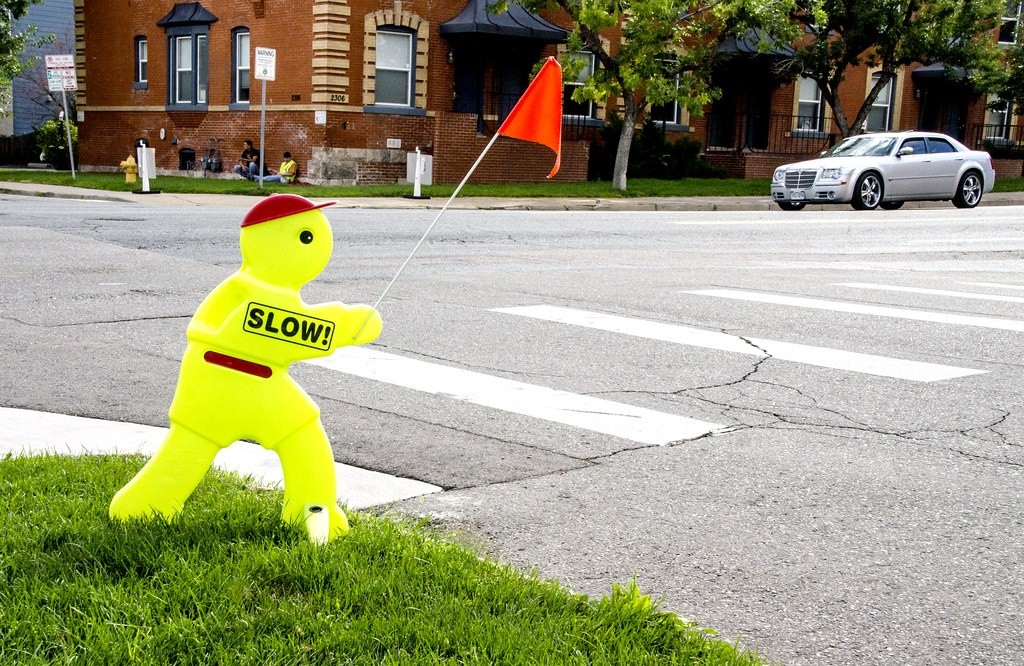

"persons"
[234,140,260,181]
[253,152,298,184]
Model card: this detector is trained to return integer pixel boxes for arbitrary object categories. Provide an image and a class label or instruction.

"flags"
[495,56,563,179]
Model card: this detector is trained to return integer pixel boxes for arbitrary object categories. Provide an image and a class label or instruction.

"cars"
[770,129,996,210]
